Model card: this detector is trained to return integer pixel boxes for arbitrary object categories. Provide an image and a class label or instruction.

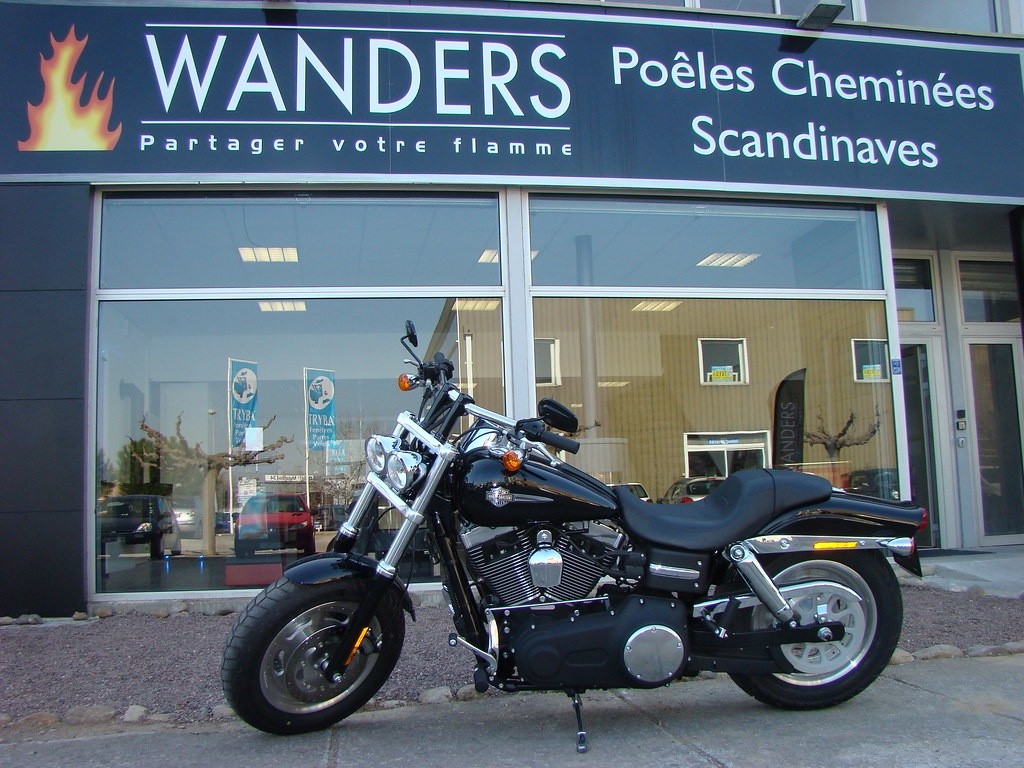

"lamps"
[796,0,846,30]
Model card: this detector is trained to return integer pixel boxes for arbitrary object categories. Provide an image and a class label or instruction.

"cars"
[606,482,655,504]
[656,476,726,504]
[97,495,181,560]
[167,494,203,541]
[839,468,900,501]
[234,494,319,559]
[214,510,237,533]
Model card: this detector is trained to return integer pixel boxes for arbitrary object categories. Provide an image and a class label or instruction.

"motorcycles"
[219,321,928,754]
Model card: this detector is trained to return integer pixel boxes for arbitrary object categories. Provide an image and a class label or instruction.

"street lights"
[208,409,216,454]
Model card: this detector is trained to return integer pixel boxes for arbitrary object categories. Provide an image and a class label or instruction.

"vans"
[315,503,345,531]
